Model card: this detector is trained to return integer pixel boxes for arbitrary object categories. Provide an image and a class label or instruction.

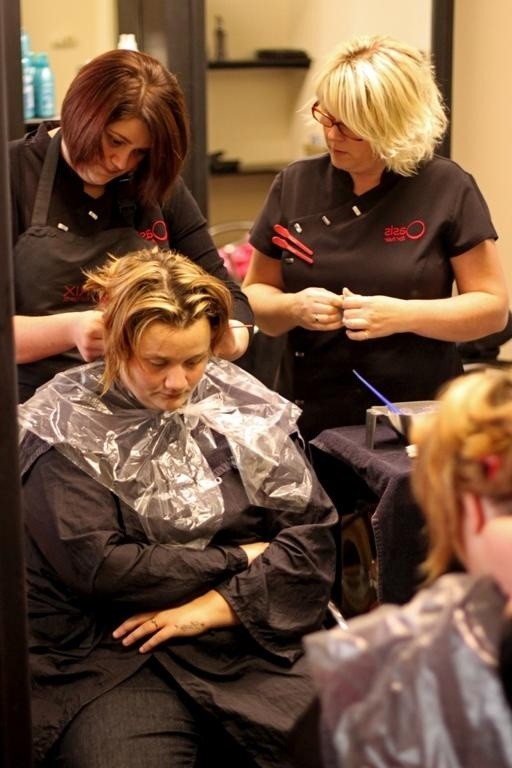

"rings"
[149,617,160,630]
[314,312,320,322]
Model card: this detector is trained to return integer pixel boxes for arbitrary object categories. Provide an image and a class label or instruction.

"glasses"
[312,100,364,142]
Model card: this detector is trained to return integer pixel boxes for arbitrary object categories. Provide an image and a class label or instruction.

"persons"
[14,244,364,765]
[2,46,259,402]
[239,28,510,571]
[309,367,509,765]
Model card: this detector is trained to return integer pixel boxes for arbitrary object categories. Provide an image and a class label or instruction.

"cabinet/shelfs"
[207,51,320,179]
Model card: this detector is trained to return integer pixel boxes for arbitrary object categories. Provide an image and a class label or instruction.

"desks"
[315,410,466,608]
